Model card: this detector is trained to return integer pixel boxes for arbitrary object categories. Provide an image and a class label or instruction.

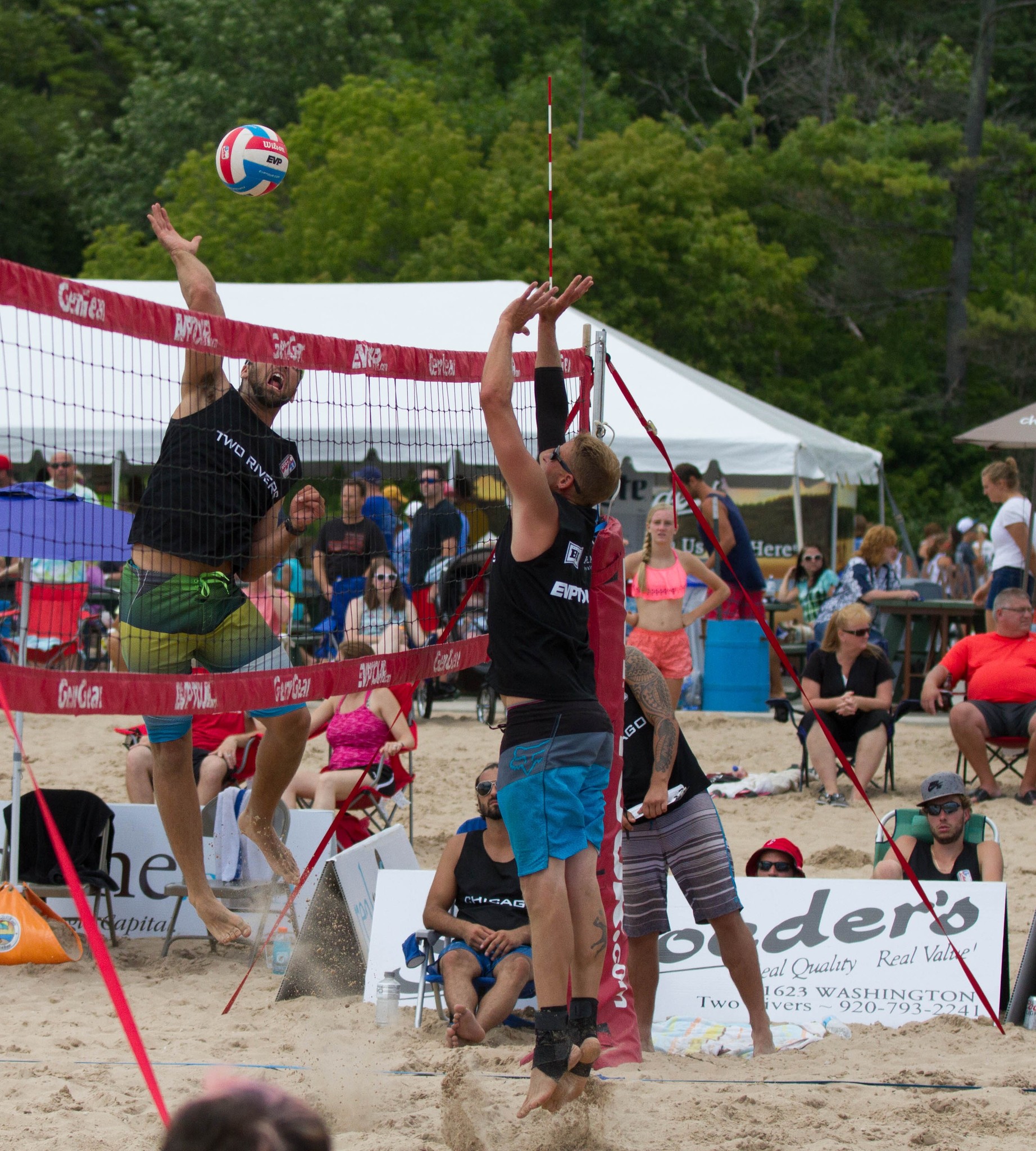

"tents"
[0,280,881,582]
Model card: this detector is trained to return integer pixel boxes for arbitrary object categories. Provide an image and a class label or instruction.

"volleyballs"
[215,124,289,196]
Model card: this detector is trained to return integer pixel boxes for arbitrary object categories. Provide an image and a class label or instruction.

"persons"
[747,835,807,879]
[878,773,1004,888]
[117,202,326,945]
[162,1078,333,1151]
[604,635,773,1056]
[424,764,535,1045]
[476,274,613,1111]
[0,450,1036,811]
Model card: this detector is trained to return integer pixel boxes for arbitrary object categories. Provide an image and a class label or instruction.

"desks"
[866,586,984,695]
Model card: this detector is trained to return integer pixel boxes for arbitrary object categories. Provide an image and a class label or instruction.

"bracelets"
[285,517,308,536]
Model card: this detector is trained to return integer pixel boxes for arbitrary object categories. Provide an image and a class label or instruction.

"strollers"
[415,545,501,726]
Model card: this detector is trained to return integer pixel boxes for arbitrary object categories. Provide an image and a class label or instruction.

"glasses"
[550,444,580,496]
[1001,606,1035,614]
[802,554,823,562]
[843,627,870,637]
[419,477,439,484]
[476,779,497,796]
[926,802,961,817]
[374,573,397,582]
[49,461,75,469]
[758,861,790,872]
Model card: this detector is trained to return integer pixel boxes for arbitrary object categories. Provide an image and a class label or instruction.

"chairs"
[413,890,537,1037]
[310,577,437,718]
[947,729,1030,796]
[162,787,302,970]
[316,682,422,849]
[0,570,89,682]
[762,688,901,789]
[0,788,118,948]
[754,552,1004,718]
[868,807,1000,883]
[115,721,266,786]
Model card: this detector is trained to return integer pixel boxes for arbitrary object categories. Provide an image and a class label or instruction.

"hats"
[0,454,12,471]
[915,770,971,807]
[956,516,978,534]
[746,837,806,878]
[352,466,384,484]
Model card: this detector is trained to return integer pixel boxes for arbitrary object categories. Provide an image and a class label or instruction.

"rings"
[228,754,232,756]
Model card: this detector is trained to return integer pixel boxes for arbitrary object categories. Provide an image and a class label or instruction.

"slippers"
[964,784,1006,802]
[1014,790,1036,806]
[817,791,848,808]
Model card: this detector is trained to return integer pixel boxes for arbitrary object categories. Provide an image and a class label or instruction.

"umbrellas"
[953,402,1036,450]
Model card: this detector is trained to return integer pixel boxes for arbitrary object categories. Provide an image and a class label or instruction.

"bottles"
[375,971,401,1028]
[732,765,748,779]
[272,927,290,975]
[766,575,776,602]
